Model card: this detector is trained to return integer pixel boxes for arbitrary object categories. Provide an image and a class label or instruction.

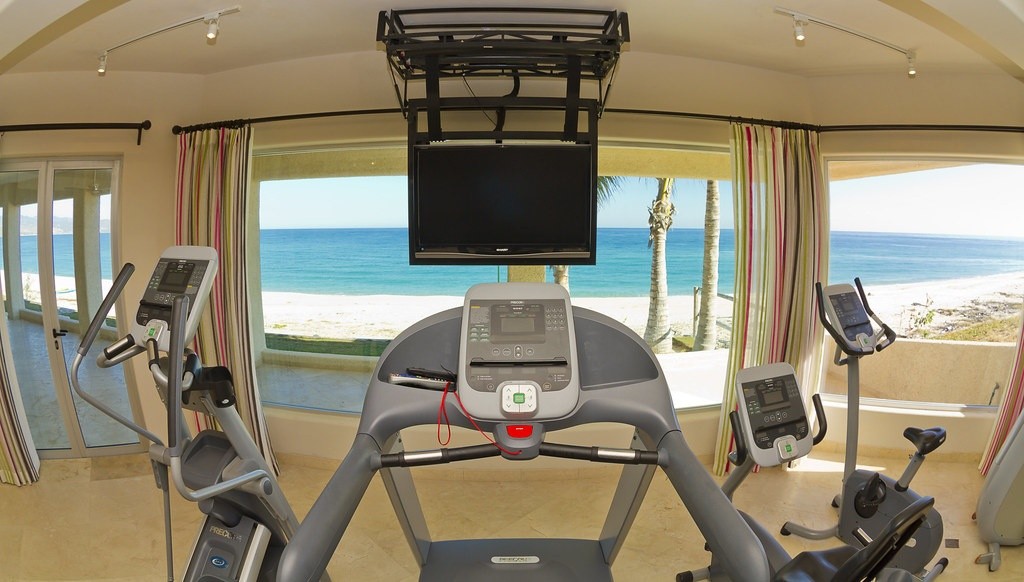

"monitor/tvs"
[413,144,592,259]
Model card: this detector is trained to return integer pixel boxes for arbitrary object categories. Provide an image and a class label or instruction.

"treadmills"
[273,281,772,582]
